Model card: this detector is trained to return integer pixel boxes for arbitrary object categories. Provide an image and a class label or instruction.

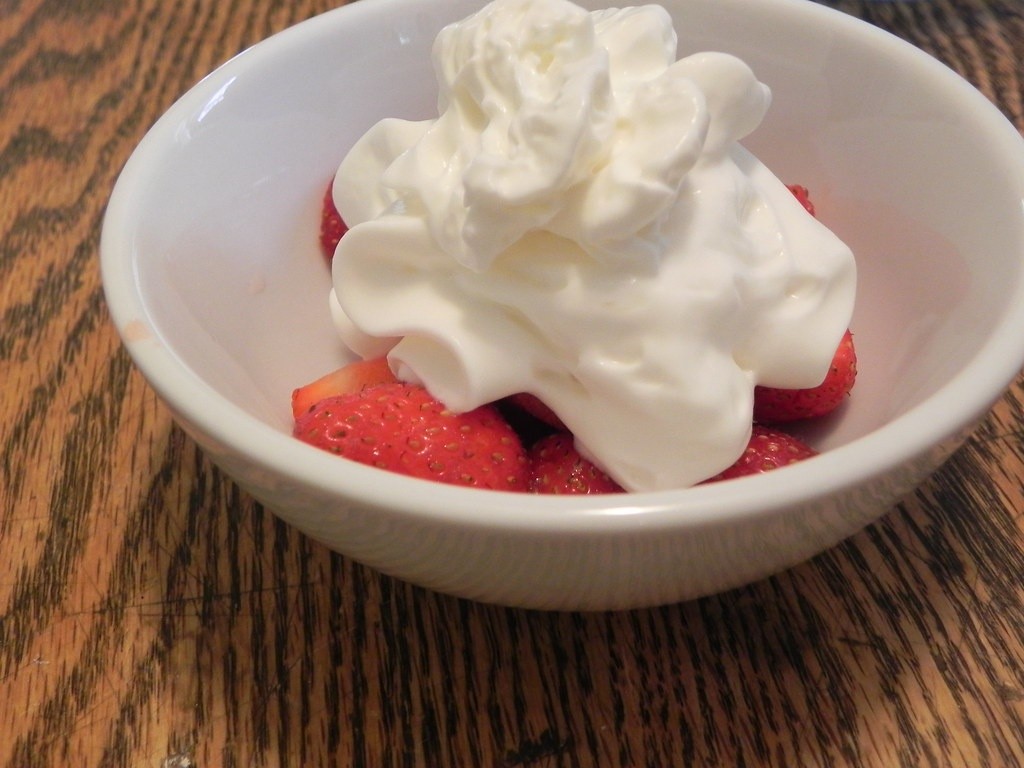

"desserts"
[325,0,858,495]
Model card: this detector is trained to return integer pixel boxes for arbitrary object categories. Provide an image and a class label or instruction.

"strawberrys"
[288,179,858,496]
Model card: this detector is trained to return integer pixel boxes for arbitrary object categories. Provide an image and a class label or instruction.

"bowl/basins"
[99,0,1024,611]
